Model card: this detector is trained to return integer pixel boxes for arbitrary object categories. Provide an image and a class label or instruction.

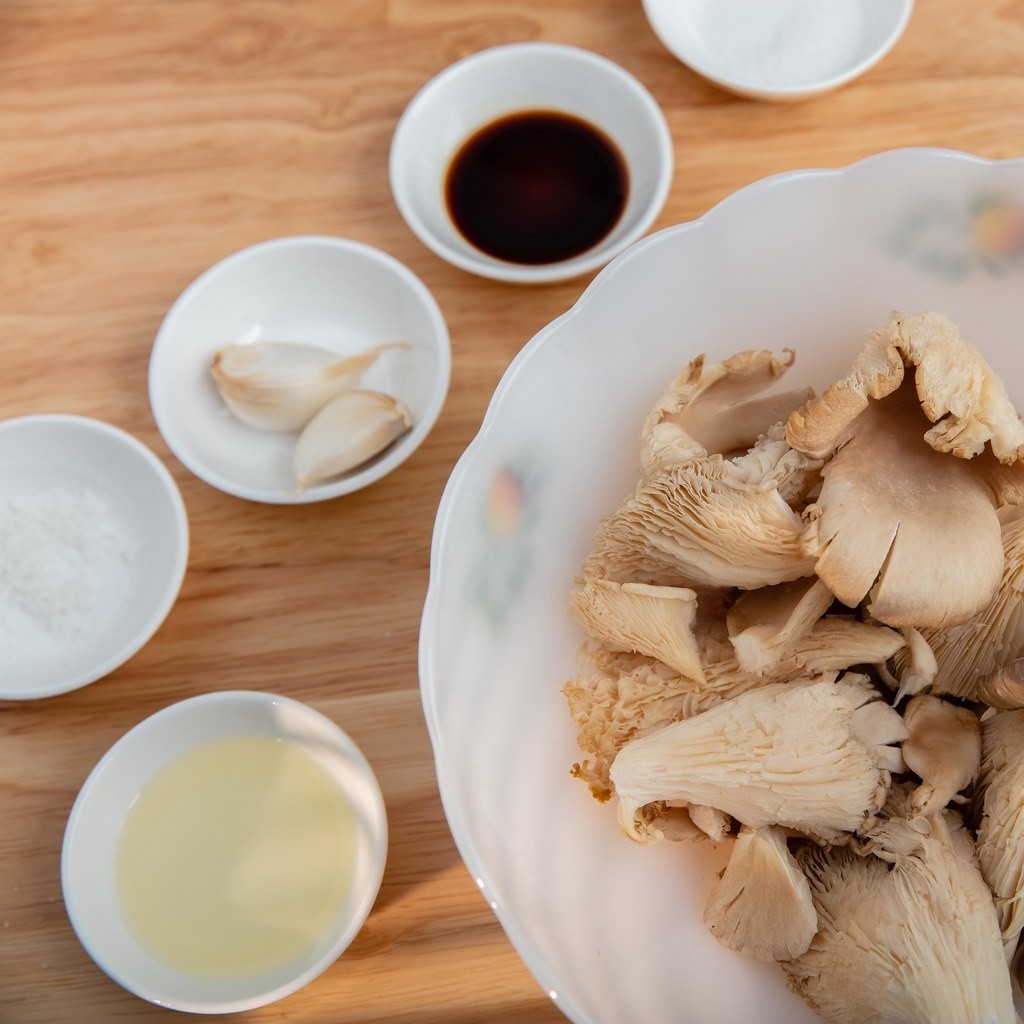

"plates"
[641,0,914,103]
[147,235,452,505]
[0,414,189,701]
[418,142,1024,1024]
[389,41,673,283]
[60,689,388,1014]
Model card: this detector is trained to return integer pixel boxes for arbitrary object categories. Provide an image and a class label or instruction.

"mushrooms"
[562,306,1024,1023]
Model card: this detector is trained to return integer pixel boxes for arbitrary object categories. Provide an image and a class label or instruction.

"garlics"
[212,336,414,488]
